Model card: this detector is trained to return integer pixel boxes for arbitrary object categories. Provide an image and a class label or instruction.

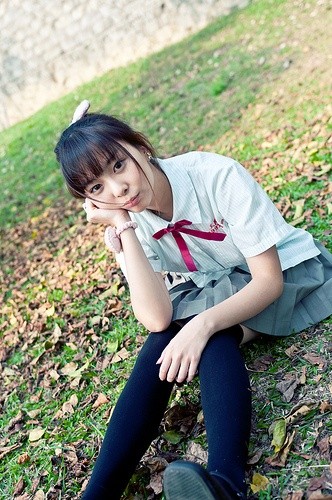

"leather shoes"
[163,460,246,500]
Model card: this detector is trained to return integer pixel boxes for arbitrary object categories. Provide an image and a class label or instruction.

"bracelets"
[104,221,138,254]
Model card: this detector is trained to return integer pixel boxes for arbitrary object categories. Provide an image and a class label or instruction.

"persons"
[54,100,332,500]
